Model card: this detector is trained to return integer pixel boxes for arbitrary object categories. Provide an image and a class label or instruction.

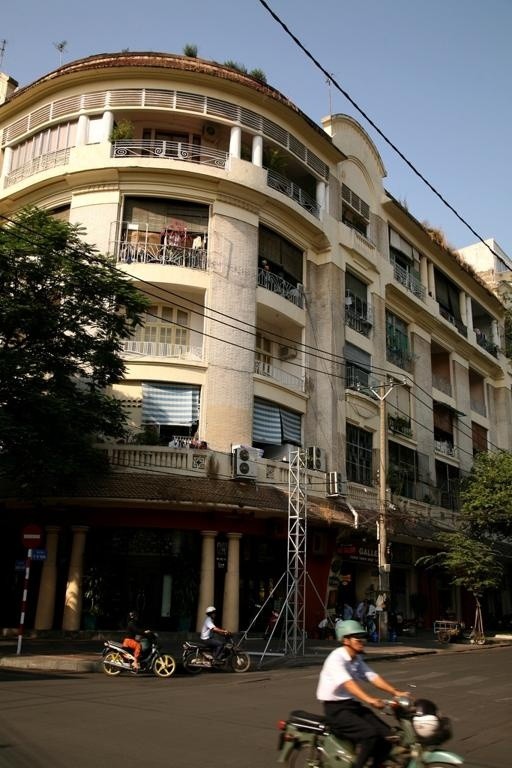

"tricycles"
[431,619,486,646]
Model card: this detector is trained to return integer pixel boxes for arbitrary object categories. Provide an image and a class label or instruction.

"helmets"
[127,611,136,619]
[415,699,436,716]
[335,620,365,642]
[206,606,216,613]
[413,714,438,737]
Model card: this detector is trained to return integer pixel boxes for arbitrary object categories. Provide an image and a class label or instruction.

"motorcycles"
[276,685,469,768]
[182,630,253,676]
[100,630,177,680]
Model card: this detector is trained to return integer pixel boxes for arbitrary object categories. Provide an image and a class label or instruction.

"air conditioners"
[308,446,327,472]
[232,445,257,480]
[325,472,344,498]
[280,345,297,360]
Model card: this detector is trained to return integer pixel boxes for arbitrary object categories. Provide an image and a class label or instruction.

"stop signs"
[19,524,46,550]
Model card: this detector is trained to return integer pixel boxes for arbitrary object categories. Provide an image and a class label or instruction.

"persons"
[200,607,228,660]
[316,620,416,768]
[121,612,151,668]
[317,599,381,640]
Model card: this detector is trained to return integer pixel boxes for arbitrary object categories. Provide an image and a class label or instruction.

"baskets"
[413,718,452,745]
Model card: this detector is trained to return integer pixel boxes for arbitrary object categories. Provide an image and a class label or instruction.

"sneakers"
[132,663,141,669]
[212,660,225,665]
